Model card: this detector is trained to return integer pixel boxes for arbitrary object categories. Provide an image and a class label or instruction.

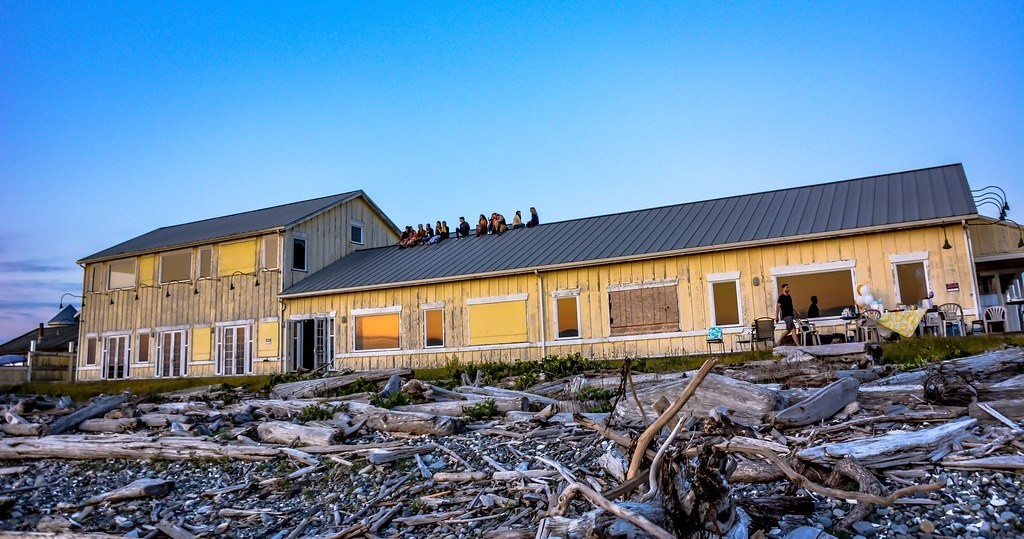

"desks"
[1005,298,1024,331]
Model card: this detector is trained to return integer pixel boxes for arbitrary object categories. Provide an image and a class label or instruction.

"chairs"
[706,301,1009,355]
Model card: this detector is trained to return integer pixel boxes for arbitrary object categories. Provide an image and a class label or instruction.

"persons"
[475,214,487,237]
[400,226,416,249]
[526,207,540,228]
[418,224,434,246]
[410,224,425,248]
[425,221,442,246]
[435,221,449,244]
[455,217,470,240]
[775,284,801,347]
[487,213,509,235]
[512,211,525,229]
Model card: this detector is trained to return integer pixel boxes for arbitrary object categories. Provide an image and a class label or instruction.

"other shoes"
[487,230,492,234]
[496,230,502,235]
[456,236,463,238]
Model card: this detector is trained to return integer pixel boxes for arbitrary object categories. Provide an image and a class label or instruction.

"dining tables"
[838,307,946,343]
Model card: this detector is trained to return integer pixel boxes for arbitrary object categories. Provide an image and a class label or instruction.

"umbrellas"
[0,355,27,367]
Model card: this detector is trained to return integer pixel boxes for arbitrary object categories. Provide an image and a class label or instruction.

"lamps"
[59,267,281,309]
[942,186,1024,249]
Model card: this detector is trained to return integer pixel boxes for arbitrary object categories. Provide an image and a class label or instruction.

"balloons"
[856,285,874,309]
